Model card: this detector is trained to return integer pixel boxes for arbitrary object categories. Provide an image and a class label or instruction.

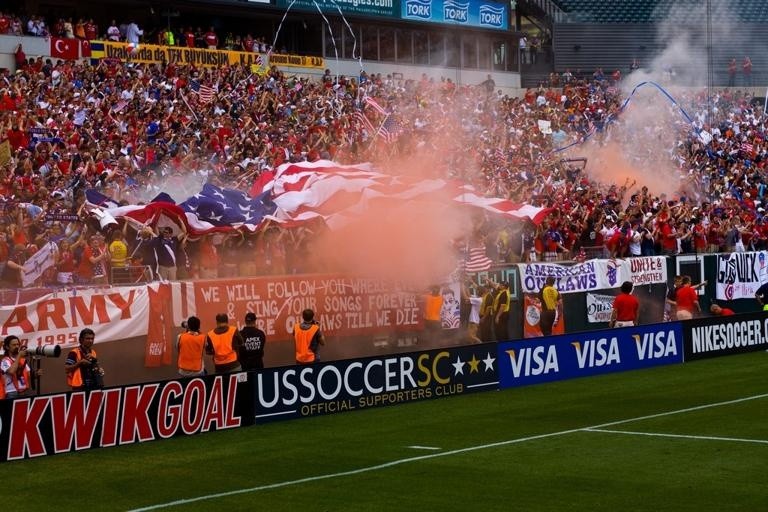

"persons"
[0,10,768,400]
